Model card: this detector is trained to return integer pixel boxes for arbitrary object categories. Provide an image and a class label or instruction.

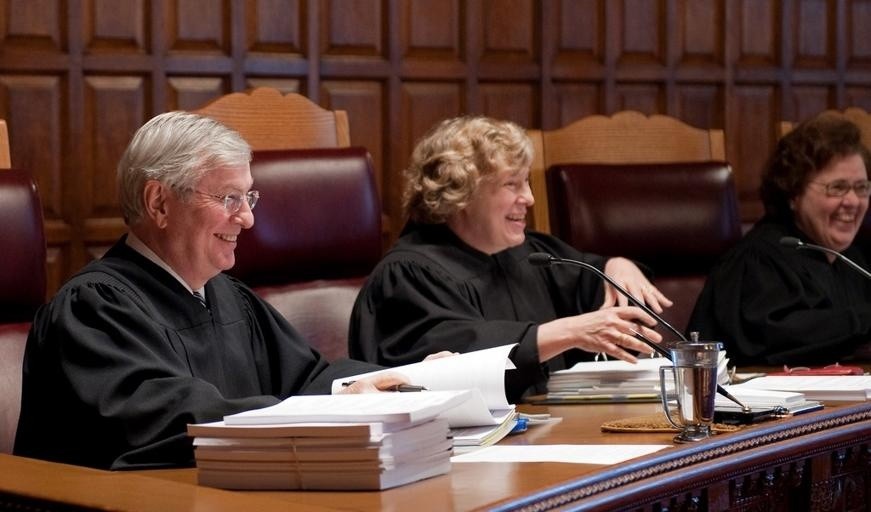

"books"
[186,340,551,490]
[546,350,734,405]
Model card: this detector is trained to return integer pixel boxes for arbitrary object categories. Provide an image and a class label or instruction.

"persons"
[346,111,673,404]
[682,117,871,366]
[11,112,460,471]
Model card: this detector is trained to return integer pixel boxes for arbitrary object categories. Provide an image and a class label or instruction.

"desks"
[0,366,871,512]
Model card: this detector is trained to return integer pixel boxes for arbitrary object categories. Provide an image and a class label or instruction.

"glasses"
[802,178,871,200]
[166,183,262,215]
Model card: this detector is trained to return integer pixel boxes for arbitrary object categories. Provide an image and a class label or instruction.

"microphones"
[527,252,776,425]
[779,236,871,280]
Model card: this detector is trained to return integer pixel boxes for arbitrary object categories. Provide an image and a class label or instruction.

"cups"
[651,340,726,441]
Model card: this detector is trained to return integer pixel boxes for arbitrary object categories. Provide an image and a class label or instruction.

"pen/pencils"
[342,381,432,392]
[630,327,744,407]
